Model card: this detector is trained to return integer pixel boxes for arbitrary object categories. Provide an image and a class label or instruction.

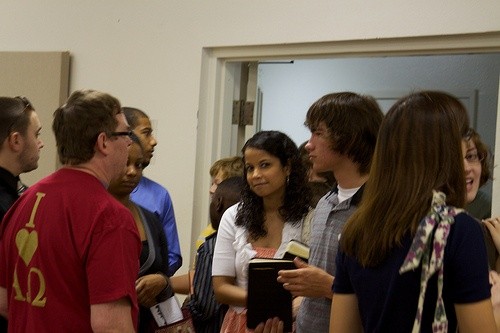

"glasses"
[7,95,32,137]
[110,130,134,140]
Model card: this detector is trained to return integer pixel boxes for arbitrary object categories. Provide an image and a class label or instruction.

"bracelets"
[155,271,171,302]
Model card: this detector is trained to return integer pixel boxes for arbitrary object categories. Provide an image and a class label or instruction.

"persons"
[179,176,244,333]
[119,107,183,278]
[170,157,244,319]
[459,127,500,325]
[0,95,44,333]
[108,131,174,333]
[0,90,142,333]
[330,89,499,332]
[211,131,316,333]
[296,140,331,208]
[246,92,385,333]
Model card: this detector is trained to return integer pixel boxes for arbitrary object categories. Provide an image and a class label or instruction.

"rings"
[492,220,497,225]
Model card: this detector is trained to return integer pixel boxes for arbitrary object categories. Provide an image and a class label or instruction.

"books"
[281,238,311,263]
[245,255,295,333]
